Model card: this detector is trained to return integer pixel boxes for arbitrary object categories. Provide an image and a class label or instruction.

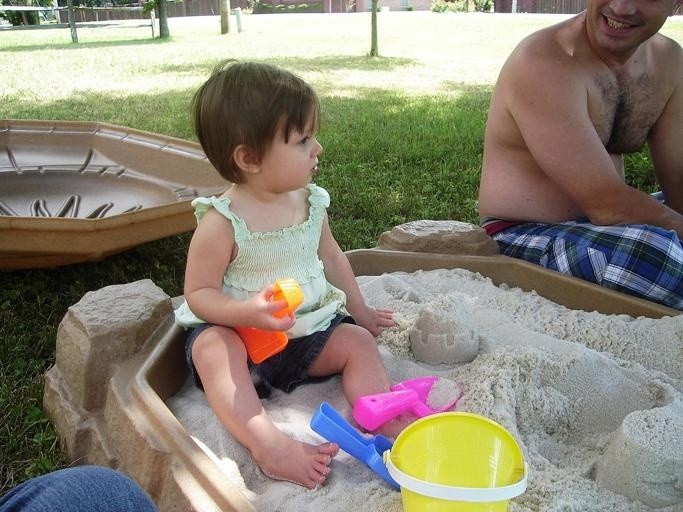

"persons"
[182,60,419,490]
[475,0,681,310]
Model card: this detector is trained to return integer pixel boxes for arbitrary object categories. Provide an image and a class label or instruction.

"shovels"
[310,403,401,494]
[352,377,464,430]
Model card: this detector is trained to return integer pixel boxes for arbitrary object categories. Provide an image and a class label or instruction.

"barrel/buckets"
[384,411,531,511]
